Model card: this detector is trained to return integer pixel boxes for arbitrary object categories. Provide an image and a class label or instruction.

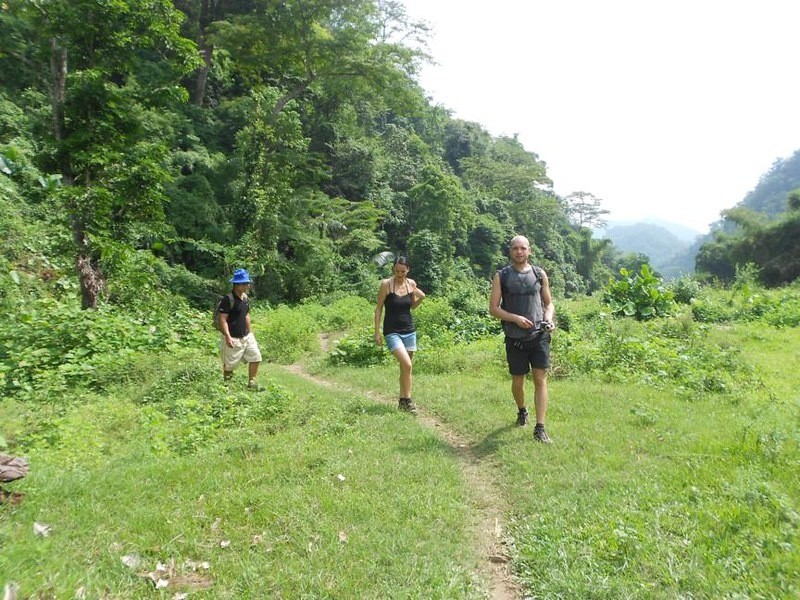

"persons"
[217,269,266,392]
[374,256,426,416]
[489,234,555,444]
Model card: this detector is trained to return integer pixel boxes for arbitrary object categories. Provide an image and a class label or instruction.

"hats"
[230,269,252,283]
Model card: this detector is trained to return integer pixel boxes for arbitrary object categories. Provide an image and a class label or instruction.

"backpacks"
[212,293,247,334]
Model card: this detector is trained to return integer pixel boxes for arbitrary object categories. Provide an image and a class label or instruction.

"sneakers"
[405,398,418,416]
[398,398,406,413]
[246,383,266,391]
[515,408,529,427]
[533,423,553,443]
